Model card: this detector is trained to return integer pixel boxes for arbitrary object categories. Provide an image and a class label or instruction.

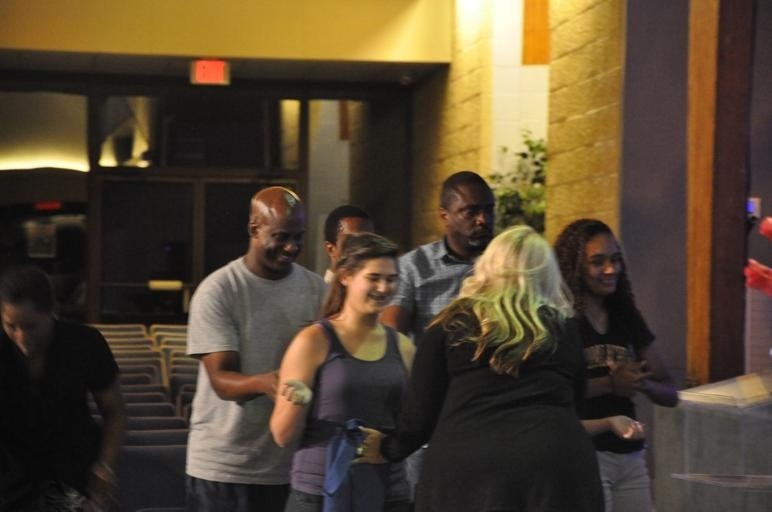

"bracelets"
[93,460,117,479]
[609,374,617,396]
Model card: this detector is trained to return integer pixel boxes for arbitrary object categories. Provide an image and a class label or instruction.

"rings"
[354,443,366,458]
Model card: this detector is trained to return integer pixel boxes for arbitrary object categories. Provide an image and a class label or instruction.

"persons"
[0,272,128,511]
[347,225,607,510]
[324,206,379,279]
[380,172,497,502]
[554,218,679,511]
[182,184,330,510]
[269,229,425,510]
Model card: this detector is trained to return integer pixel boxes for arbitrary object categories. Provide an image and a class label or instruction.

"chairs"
[76,319,213,512]
